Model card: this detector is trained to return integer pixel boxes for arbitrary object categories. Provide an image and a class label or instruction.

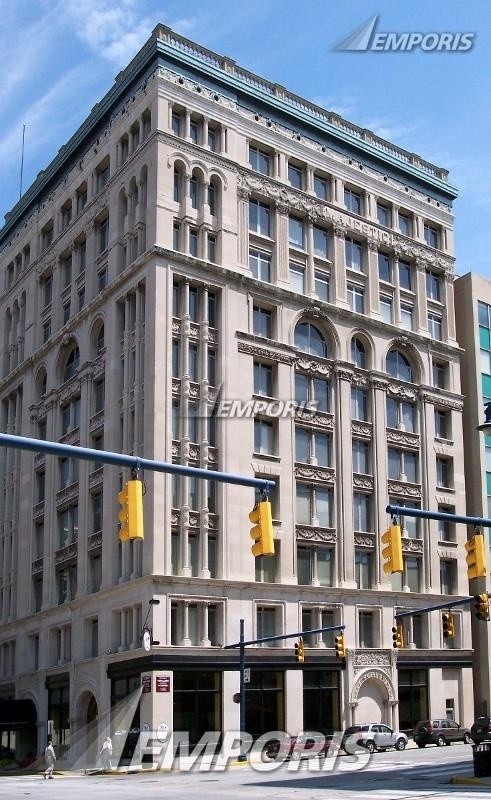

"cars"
[265,734,340,762]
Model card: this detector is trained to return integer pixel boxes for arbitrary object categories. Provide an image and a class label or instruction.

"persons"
[41,740,56,780]
[99,735,112,771]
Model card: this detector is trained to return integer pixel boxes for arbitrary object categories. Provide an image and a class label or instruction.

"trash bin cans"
[470,743,491,778]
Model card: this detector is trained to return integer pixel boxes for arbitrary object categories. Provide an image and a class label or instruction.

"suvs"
[471,717,491,744]
[341,722,408,756]
[412,719,471,748]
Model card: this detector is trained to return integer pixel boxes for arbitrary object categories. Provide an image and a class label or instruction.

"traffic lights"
[473,592,491,622]
[248,500,275,559]
[392,624,405,650]
[464,533,487,583]
[117,479,144,544]
[380,524,404,574]
[441,611,455,639]
[335,632,347,659]
[294,639,304,664]
